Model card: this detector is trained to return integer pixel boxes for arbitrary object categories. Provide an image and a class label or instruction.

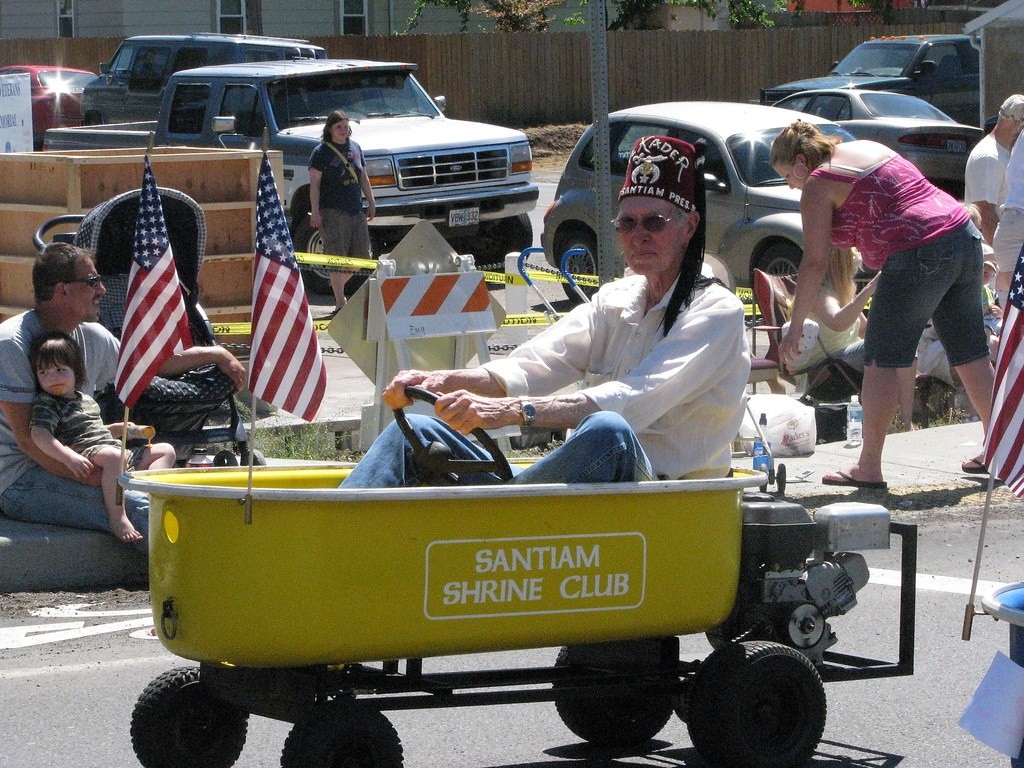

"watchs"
[517,396,537,435]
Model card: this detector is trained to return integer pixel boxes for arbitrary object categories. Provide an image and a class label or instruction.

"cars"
[0,64,101,152]
[760,31,981,127]
[535,99,882,304]
[770,89,984,200]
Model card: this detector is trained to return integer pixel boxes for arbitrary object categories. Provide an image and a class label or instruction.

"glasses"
[45,274,101,288]
[610,210,682,234]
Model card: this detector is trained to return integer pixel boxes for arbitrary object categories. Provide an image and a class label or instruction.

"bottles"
[846,395,862,446]
[186,448,214,468]
[753,413,772,471]
[128,425,155,439]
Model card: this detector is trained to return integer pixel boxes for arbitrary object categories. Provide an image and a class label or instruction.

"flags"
[981,239,1024,500]
[114,155,189,409]
[246,153,327,422]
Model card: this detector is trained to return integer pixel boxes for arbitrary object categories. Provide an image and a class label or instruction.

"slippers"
[962,459,988,474]
[822,471,887,488]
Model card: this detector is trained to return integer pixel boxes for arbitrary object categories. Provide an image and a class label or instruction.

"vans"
[81,31,328,128]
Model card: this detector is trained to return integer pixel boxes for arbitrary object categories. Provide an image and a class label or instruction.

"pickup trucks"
[39,59,539,307]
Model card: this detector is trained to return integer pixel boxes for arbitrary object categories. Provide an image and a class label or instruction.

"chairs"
[938,54,962,72]
[821,99,846,121]
[752,267,930,430]
[618,251,778,460]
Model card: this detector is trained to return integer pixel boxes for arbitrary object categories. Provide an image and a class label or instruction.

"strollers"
[517,246,788,496]
[32,188,266,466]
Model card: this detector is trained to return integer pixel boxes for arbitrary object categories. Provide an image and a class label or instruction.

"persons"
[0,242,245,555]
[25,331,176,540]
[769,95,1024,488]
[307,112,375,315]
[336,136,749,488]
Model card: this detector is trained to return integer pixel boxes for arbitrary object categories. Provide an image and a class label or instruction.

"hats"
[617,136,697,212]
[998,94,1024,120]
